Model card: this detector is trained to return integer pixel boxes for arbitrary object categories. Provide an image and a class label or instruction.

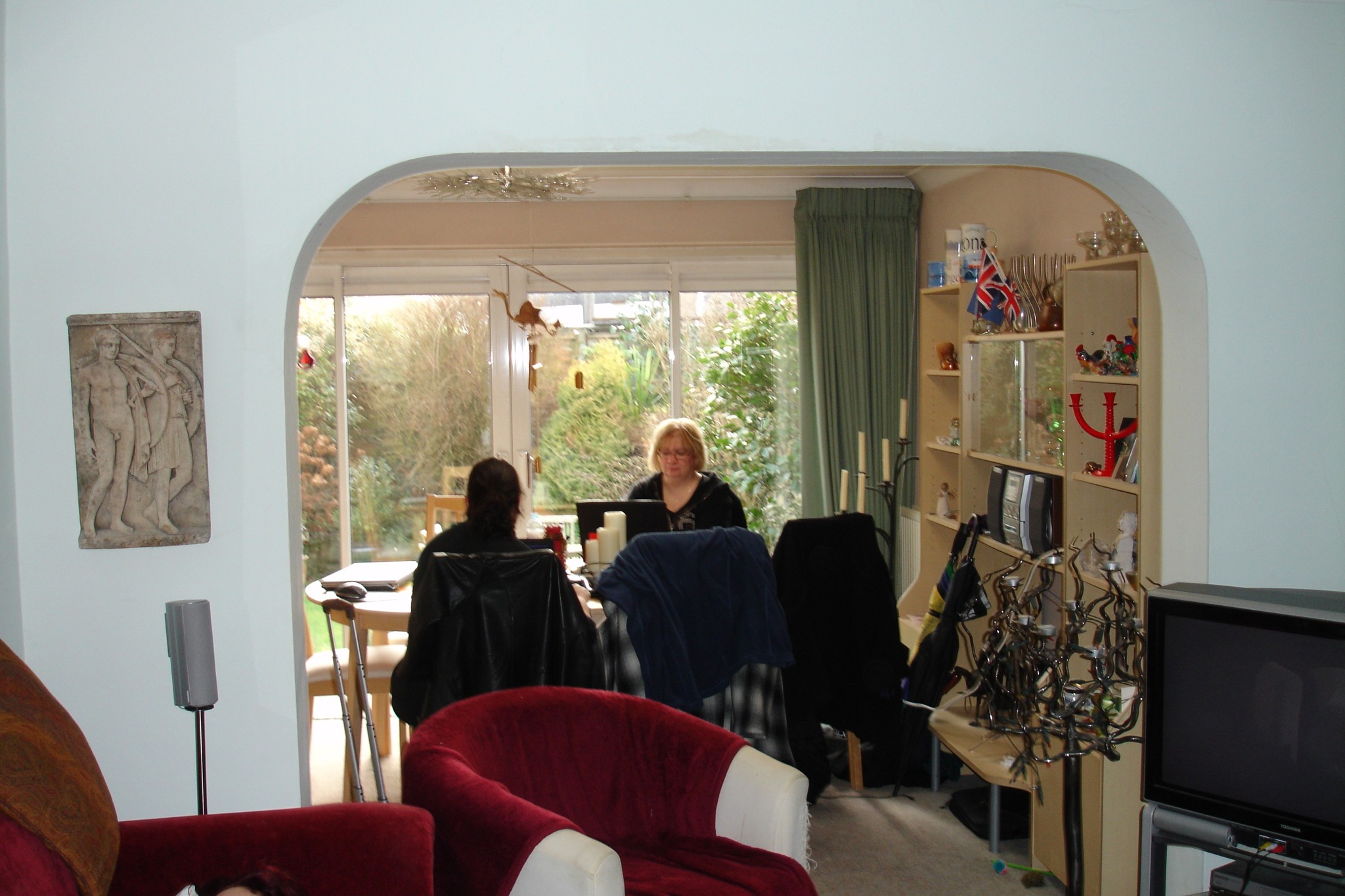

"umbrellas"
[893,512,992,797]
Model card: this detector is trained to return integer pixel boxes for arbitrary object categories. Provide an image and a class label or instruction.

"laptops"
[321,560,419,592]
[575,498,673,562]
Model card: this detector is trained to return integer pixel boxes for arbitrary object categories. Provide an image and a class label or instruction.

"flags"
[966,247,1023,327]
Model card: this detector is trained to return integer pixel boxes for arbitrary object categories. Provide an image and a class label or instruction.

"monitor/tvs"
[1138,581,1345,881]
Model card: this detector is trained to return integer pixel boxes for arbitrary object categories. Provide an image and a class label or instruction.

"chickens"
[1075,345,1110,375]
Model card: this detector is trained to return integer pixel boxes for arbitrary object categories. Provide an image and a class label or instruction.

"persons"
[390,458,537,727]
[78,328,193,537]
[628,419,748,531]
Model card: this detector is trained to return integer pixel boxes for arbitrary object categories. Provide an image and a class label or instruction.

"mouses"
[337,581,368,600]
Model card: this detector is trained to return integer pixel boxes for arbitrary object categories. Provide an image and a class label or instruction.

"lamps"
[413,167,598,202]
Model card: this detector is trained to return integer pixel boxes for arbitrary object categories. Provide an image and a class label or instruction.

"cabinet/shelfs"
[894,251,1163,896]
[1136,802,1345,896]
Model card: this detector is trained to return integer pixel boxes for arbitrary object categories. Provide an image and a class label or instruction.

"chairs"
[393,495,896,789]
[304,609,409,798]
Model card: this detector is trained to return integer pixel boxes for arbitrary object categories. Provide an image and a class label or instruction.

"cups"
[584,511,626,576]
[1131,231,1147,253]
[960,224,997,282]
[928,262,944,288]
[944,229,962,286]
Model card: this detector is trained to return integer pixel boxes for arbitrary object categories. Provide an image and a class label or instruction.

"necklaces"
[662,473,696,508]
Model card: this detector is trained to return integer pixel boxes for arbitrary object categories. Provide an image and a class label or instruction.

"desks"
[305,577,610,804]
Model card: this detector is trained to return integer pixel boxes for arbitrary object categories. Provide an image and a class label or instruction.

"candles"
[604,512,627,553]
[596,525,620,563]
[839,398,907,511]
[587,540,599,572]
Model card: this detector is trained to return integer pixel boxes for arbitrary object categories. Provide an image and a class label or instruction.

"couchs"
[399,686,821,896]
[0,639,433,896]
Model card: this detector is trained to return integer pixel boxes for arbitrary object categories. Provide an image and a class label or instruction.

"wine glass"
[1076,210,1132,260]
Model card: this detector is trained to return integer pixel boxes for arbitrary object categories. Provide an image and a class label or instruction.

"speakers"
[162,599,219,712]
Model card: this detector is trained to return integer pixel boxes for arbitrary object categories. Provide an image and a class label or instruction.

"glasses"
[657,449,690,460]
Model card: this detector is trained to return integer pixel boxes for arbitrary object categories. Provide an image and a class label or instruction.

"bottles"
[948,418,959,438]
[527,525,567,570]
